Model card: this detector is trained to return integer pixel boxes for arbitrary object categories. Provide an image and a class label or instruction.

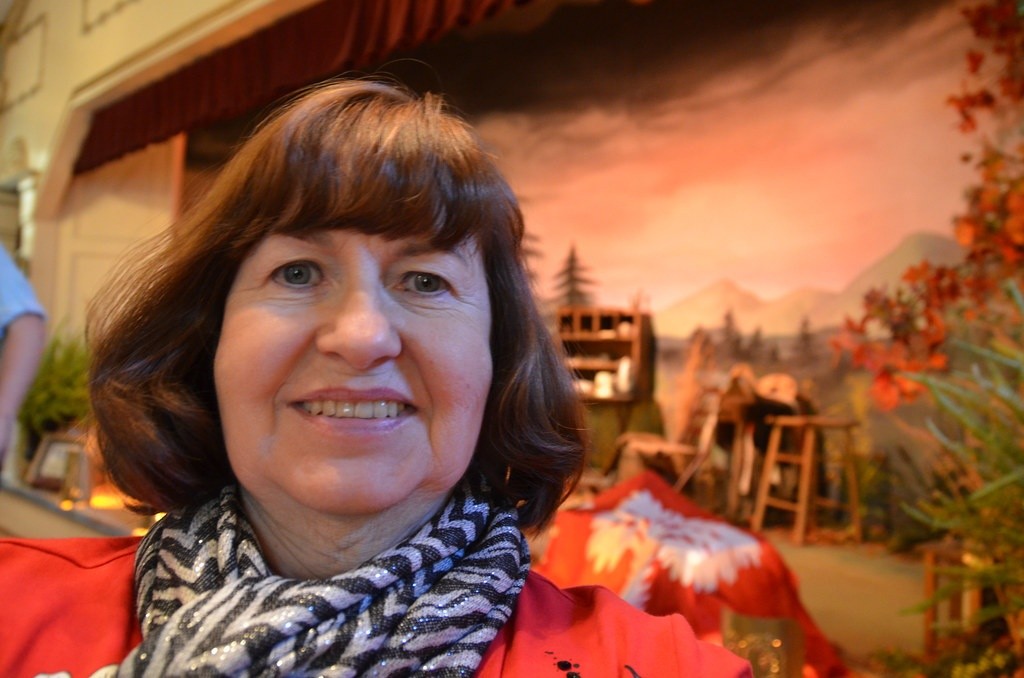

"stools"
[750,416,864,546]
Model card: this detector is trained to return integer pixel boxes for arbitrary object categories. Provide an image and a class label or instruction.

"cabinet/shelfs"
[555,307,655,405]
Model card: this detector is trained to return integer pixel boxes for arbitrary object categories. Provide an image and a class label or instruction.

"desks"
[723,404,758,521]
[923,544,963,654]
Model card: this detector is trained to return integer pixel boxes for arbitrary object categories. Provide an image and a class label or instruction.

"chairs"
[618,378,723,514]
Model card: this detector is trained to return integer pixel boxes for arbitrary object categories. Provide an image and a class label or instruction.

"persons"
[0,240,49,470]
[0,79,754,678]
